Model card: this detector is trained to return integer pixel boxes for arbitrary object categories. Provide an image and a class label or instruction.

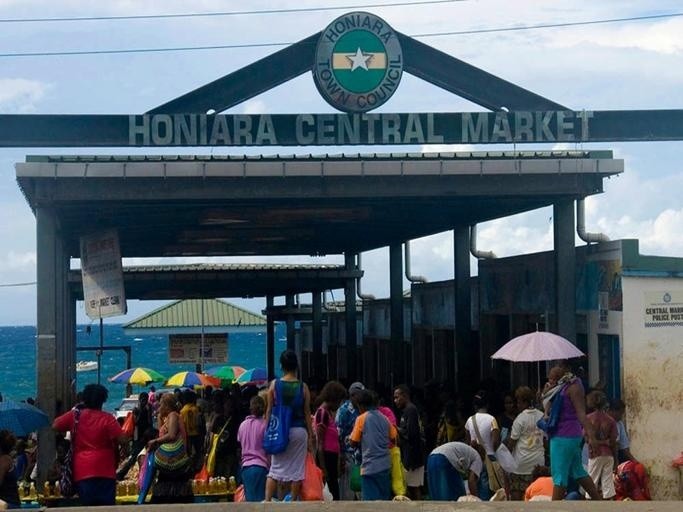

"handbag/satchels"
[261,404,292,455]
[206,432,217,475]
[390,446,408,498]
[349,463,362,492]
[58,445,75,497]
[154,439,191,476]
[120,412,134,437]
[486,460,506,491]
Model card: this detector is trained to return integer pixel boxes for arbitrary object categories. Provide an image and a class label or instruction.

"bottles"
[193,476,236,494]
[117,480,152,496]
[17,481,62,499]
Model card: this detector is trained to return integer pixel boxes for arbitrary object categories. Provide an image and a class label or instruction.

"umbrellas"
[491,321,586,392]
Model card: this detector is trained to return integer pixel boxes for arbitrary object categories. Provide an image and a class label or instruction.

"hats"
[348,381,365,395]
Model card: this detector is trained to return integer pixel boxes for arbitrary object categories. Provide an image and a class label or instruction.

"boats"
[75,360,99,372]
